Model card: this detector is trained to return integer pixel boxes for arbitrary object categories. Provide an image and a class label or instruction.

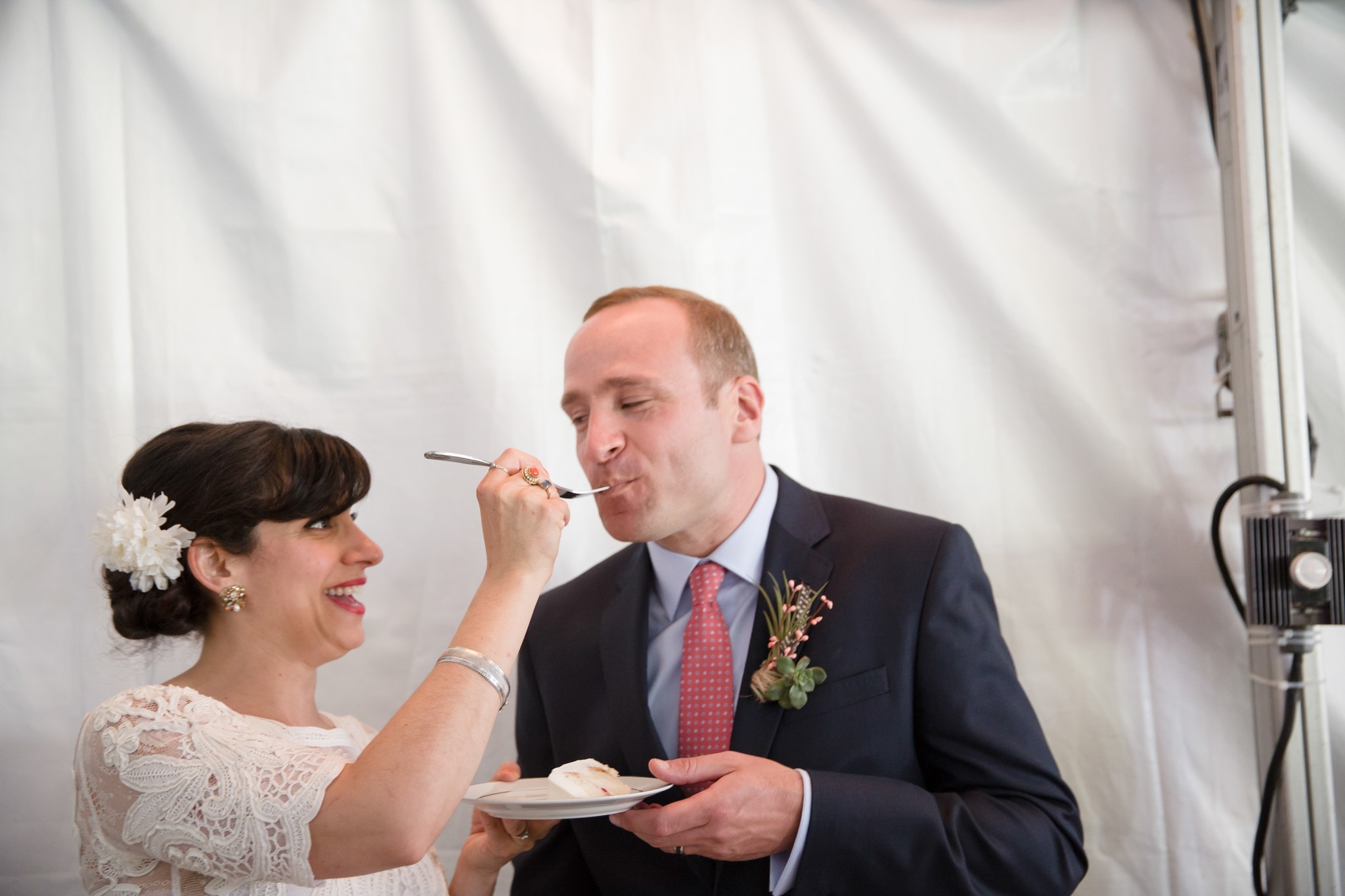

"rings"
[518,465,540,486]
[538,480,553,498]
[514,828,529,840]
[674,846,684,855]
[488,463,509,474]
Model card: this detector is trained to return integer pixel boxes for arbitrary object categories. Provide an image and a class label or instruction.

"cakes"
[548,753,630,803]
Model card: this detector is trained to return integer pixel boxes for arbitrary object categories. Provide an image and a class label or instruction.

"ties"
[676,559,735,797]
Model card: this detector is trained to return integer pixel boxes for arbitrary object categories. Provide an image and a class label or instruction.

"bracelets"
[435,647,511,712]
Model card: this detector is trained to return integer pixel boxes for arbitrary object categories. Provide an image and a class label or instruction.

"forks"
[423,448,610,499]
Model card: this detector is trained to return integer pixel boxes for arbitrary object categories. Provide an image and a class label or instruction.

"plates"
[462,775,674,821]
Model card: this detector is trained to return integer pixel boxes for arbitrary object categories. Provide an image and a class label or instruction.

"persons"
[70,421,569,896]
[507,288,1087,895]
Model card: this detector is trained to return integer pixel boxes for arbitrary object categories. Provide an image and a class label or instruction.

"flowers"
[103,483,198,593]
[743,571,833,709]
